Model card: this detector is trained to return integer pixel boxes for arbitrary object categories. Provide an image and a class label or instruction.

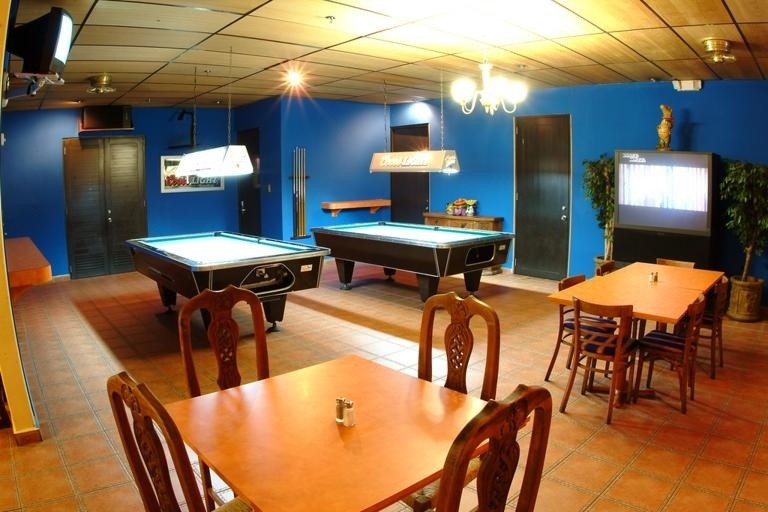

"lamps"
[368,63,460,174]
[175,47,253,178]
[450,59,528,116]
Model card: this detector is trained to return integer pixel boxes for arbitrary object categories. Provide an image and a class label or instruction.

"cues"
[294,147,308,236]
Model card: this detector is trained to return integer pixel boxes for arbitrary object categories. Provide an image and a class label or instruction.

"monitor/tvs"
[614,148,713,236]
[5,6,74,78]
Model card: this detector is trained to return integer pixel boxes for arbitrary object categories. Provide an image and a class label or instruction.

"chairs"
[398,292,501,512]
[107,371,251,512]
[178,284,269,511]
[544,258,728,426]
[437,385,552,511]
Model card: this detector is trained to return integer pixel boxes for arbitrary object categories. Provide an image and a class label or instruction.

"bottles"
[649,272,658,282]
[343,399,355,427]
[335,396,345,423]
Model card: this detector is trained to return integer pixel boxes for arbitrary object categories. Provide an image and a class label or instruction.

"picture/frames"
[161,156,225,193]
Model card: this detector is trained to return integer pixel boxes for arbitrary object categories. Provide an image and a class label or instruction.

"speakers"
[77,104,136,134]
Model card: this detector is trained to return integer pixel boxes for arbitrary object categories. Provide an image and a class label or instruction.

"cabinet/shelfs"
[422,212,504,276]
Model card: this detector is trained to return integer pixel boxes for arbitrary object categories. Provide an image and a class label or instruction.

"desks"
[310,221,516,311]
[126,231,330,332]
[159,355,532,512]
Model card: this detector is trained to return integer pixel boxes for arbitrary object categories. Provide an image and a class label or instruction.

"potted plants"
[718,162,768,322]
[583,153,614,275]
